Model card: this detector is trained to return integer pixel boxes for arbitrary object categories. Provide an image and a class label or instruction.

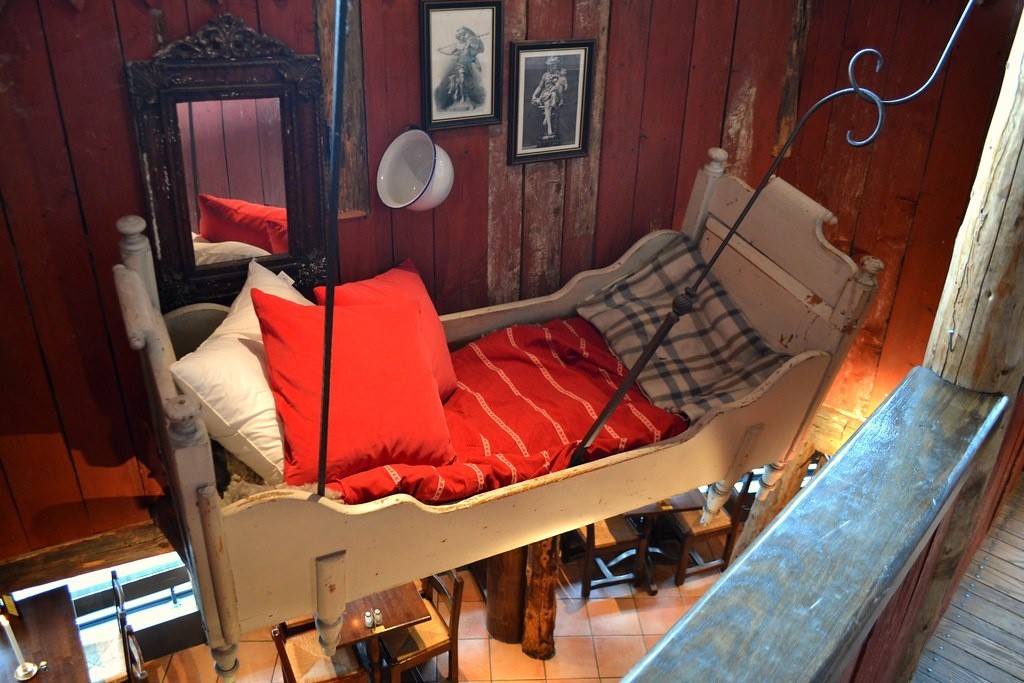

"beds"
[114,148,884,683]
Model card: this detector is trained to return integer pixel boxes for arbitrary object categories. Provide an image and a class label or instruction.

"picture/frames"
[506,37,597,167]
[419,0,504,131]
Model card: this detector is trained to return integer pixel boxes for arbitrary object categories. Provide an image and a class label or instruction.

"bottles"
[373,609,383,625]
[365,611,374,627]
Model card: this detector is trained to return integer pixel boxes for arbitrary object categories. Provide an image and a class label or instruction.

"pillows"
[313,257,459,404]
[192,233,271,265]
[168,259,316,484]
[196,192,290,255]
[249,287,459,486]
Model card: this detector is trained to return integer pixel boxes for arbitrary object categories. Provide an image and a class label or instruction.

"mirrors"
[123,13,331,310]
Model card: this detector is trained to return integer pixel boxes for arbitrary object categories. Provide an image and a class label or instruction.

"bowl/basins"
[377,125,454,211]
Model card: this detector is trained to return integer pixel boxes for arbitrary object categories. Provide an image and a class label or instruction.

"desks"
[622,488,707,596]
[0,584,91,683]
[336,581,431,683]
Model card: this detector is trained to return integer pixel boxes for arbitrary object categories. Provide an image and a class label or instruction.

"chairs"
[124,625,149,683]
[80,570,137,683]
[561,514,647,598]
[378,568,464,683]
[664,471,754,586]
[270,626,369,683]
[276,615,316,638]
